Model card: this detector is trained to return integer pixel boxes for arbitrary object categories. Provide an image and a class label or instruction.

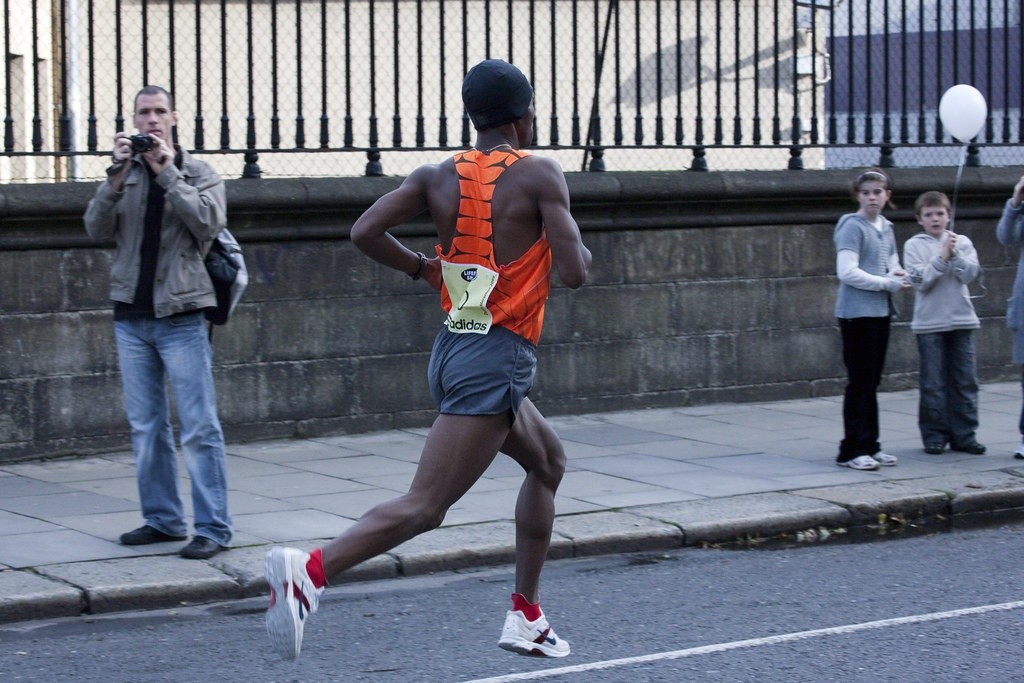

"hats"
[462,59,533,129]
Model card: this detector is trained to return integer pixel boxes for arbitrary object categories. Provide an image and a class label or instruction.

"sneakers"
[836,451,899,470]
[265,547,325,661]
[498,609,570,657]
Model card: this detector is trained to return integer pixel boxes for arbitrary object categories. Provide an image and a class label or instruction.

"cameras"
[128,135,155,154]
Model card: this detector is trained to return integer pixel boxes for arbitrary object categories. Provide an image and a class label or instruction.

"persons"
[81,84,235,563]
[904,192,986,455]
[831,170,911,469]
[263,59,592,658]
[996,174,1024,458]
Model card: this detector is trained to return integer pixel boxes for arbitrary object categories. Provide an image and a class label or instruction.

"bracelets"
[408,252,427,280]
[111,156,126,164]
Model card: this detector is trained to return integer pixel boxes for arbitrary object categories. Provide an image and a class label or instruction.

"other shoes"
[925,445,943,454]
[954,441,986,454]
[1014,444,1024,458]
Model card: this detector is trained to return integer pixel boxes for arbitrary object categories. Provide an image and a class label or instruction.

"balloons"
[939,84,987,144]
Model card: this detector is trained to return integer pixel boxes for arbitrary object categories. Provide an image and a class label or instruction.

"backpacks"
[203,225,249,327]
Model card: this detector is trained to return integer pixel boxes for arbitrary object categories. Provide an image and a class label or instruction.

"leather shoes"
[180,535,224,558]
[119,525,187,545]
